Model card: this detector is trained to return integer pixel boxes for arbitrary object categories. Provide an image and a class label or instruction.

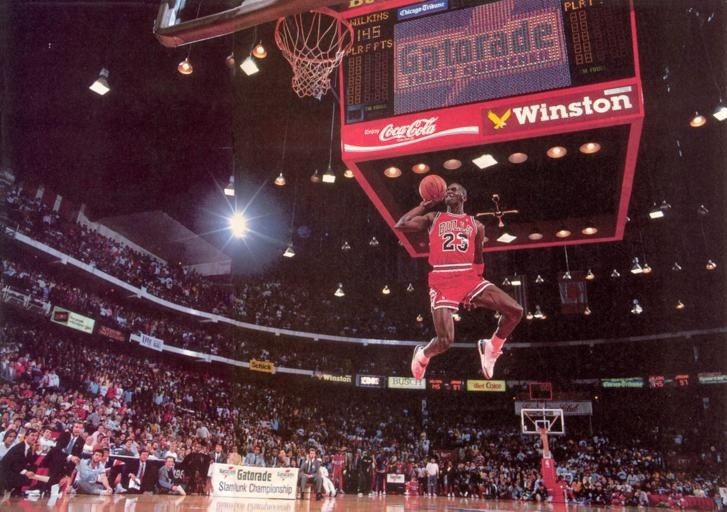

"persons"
[1,181,726,511]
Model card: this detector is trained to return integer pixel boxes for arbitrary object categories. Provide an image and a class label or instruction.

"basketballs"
[418,174,447,202]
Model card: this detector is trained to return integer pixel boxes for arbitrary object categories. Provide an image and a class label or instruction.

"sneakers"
[477,339,503,380]
[411,345,430,380]
